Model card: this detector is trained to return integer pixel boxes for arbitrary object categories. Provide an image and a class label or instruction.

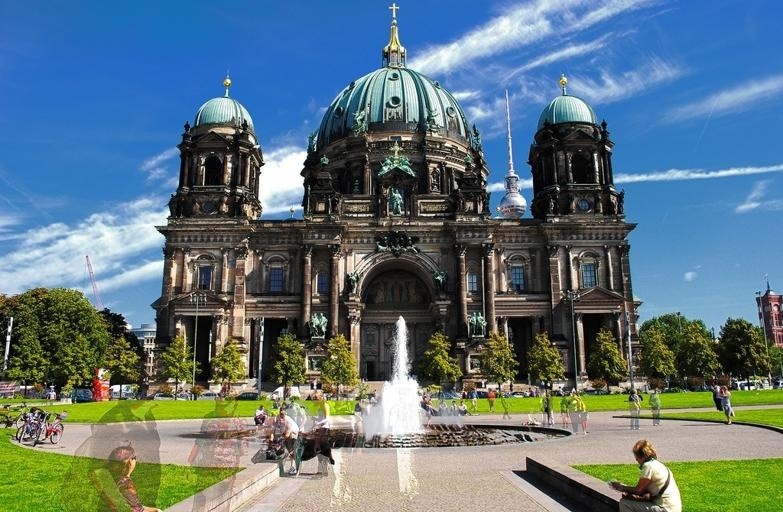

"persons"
[47,389,56,407]
[719,386,736,425]
[650,387,662,426]
[423,108,440,121]
[346,272,359,294]
[543,394,555,426]
[317,313,328,334]
[96,445,165,512]
[253,405,268,426]
[307,312,319,337]
[400,155,415,177]
[273,407,300,476]
[310,389,331,481]
[605,438,684,512]
[524,387,541,398]
[557,386,590,434]
[378,156,392,175]
[740,381,764,391]
[20,405,41,442]
[710,380,723,411]
[433,269,447,292]
[629,389,644,430]
[389,187,404,214]
[351,388,379,434]
[487,388,513,420]
[416,385,479,427]
[354,105,367,128]
[469,312,478,335]
[475,311,486,335]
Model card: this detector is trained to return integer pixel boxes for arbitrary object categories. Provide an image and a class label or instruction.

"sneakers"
[289,465,296,474]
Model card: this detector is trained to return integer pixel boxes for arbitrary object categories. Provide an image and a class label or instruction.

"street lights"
[190,290,208,400]
[559,288,581,391]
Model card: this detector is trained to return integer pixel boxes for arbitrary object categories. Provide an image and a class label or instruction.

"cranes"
[82,255,104,312]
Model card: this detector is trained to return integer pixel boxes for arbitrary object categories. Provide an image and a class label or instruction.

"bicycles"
[1,404,65,446]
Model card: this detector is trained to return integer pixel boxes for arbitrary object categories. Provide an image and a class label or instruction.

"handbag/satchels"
[621,491,654,502]
[730,407,736,417]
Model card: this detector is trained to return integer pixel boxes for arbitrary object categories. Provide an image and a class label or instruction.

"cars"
[428,385,613,399]
[640,377,783,393]
